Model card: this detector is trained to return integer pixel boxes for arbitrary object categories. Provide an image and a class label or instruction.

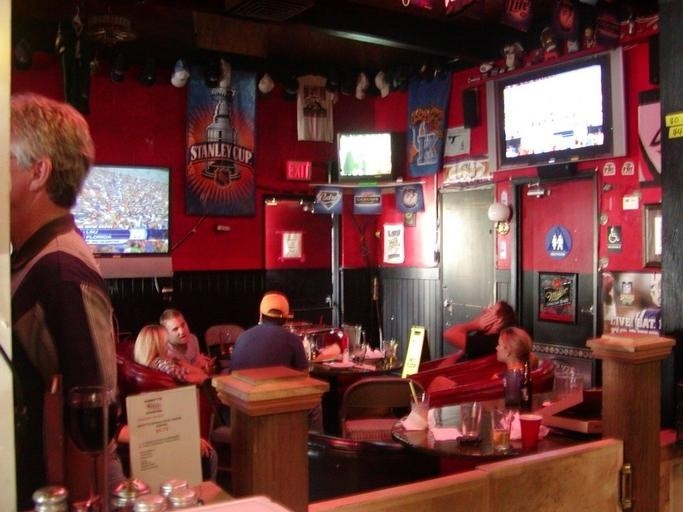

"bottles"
[520,361,531,408]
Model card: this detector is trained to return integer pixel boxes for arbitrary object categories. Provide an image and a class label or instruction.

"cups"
[460,401,483,435]
[411,393,430,421]
[492,409,515,451]
[353,344,366,363]
[520,414,542,450]
[384,341,397,369]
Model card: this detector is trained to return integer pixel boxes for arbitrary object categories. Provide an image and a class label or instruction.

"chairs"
[340,377,424,440]
[307,430,440,500]
[406,348,540,387]
[430,358,555,407]
[207,324,247,357]
[124,358,218,440]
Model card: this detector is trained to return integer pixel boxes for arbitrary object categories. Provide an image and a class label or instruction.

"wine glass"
[63,384,122,512]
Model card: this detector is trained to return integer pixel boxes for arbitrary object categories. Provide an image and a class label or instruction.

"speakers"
[649,36,659,83]
[463,87,479,129]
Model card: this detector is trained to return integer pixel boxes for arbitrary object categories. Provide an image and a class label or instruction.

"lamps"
[487,204,511,234]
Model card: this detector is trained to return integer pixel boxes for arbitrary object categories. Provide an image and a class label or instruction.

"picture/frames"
[537,271,579,326]
[641,203,663,268]
[403,212,416,227]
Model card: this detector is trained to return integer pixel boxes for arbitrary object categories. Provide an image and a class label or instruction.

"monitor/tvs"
[335,131,396,184]
[71,162,173,261]
[485,46,628,178]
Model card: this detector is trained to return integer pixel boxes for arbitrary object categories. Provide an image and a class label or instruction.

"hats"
[260,292,294,318]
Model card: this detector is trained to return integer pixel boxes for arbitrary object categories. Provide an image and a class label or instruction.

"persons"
[494,326,539,411]
[442,300,515,365]
[160,311,219,379]
[116,423,218,468]
[10,92,125,502]
[131,323,208,383]
[227,290,325,433]
